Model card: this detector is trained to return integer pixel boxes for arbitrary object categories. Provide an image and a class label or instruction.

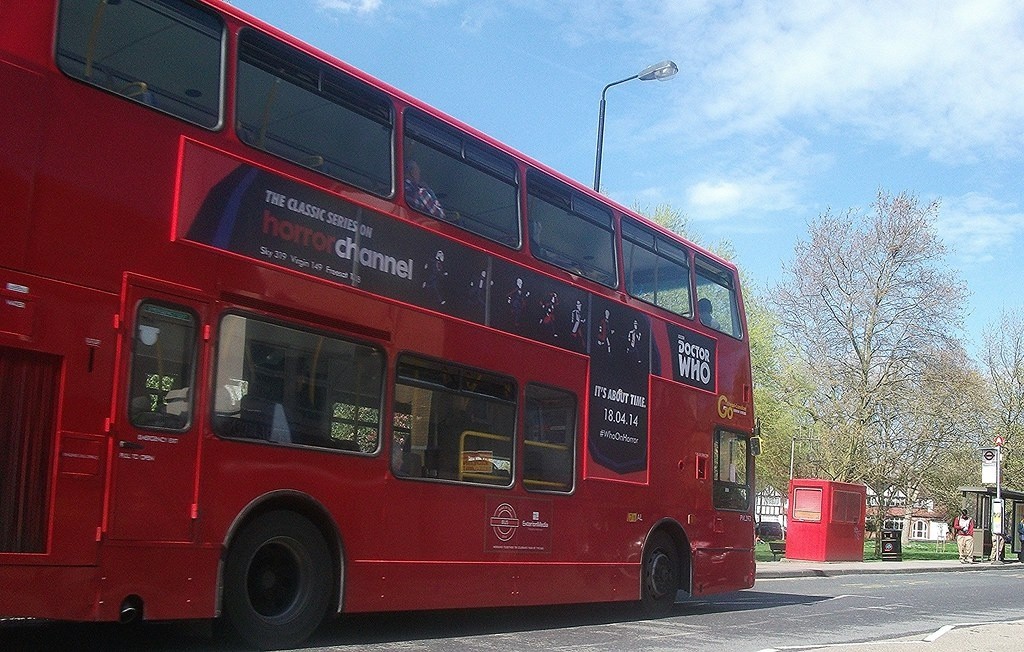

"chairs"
[241,394,290,445]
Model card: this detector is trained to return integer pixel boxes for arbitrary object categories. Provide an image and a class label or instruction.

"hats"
[962,509,967,515]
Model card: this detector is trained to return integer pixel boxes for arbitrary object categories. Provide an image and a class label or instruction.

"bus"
[0,0,763,652]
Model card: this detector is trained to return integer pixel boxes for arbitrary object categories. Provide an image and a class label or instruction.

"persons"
[987,508,1005,562]
[1017,515,1024,563]
[405,157,447,219]
[954,509,975,564]
[694,297,720,331]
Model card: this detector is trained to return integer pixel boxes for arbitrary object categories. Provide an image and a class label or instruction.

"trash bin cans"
[880,529,906,563]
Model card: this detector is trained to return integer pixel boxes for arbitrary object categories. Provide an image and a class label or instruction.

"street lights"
[592,60,680,195]
